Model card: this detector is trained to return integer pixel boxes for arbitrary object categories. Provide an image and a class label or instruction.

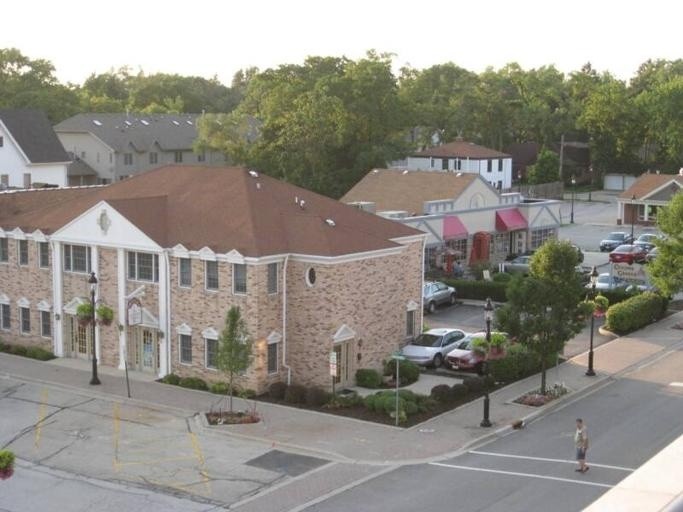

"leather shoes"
[576,467,589,472]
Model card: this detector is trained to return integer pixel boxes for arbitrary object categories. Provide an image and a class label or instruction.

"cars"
[585,272,658,293]
[399,328,516,376]
[503,256,533,276]
[423,280,457,313]
[599,232,669,265]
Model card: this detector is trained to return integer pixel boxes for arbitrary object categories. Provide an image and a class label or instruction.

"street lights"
[631,196,637,244]
[480,297,494,427]
[570,174,576,224]
[517,170,522,192]
[87,272,101,385]
[588,164,594,202]
[585,267,600,376]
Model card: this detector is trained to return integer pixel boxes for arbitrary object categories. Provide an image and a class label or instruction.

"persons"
[574,418,590,473]
[443,257,463,274]
